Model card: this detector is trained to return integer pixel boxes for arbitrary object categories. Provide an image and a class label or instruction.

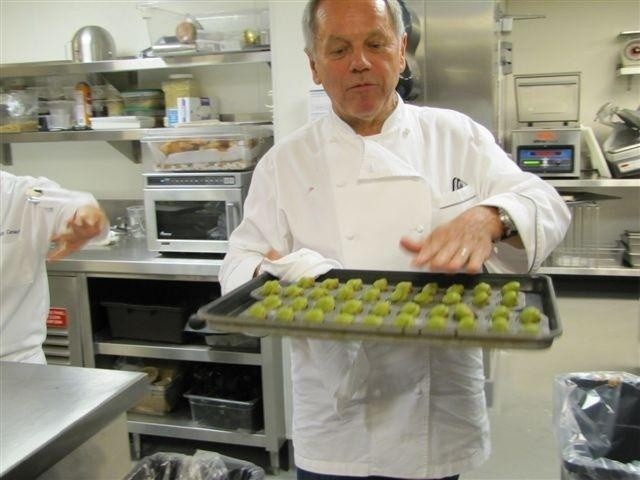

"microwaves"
[141,171,254,255]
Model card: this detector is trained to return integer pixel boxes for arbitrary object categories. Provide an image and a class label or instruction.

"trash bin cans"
[122,450,265,480]
[555,369,639,480]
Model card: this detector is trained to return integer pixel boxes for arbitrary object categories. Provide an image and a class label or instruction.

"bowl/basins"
[183,383,264,433]
[102,287,191,344]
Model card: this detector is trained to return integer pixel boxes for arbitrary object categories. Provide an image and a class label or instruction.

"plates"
[196,267,563,350]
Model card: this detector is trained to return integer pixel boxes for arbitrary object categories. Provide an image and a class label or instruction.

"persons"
[1,170,109,364]
[219,0,571,480]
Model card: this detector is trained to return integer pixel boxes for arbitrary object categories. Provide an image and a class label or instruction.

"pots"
[68,23,119,61]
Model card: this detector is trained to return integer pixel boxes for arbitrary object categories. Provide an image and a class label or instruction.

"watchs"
[498,208,516,240]
